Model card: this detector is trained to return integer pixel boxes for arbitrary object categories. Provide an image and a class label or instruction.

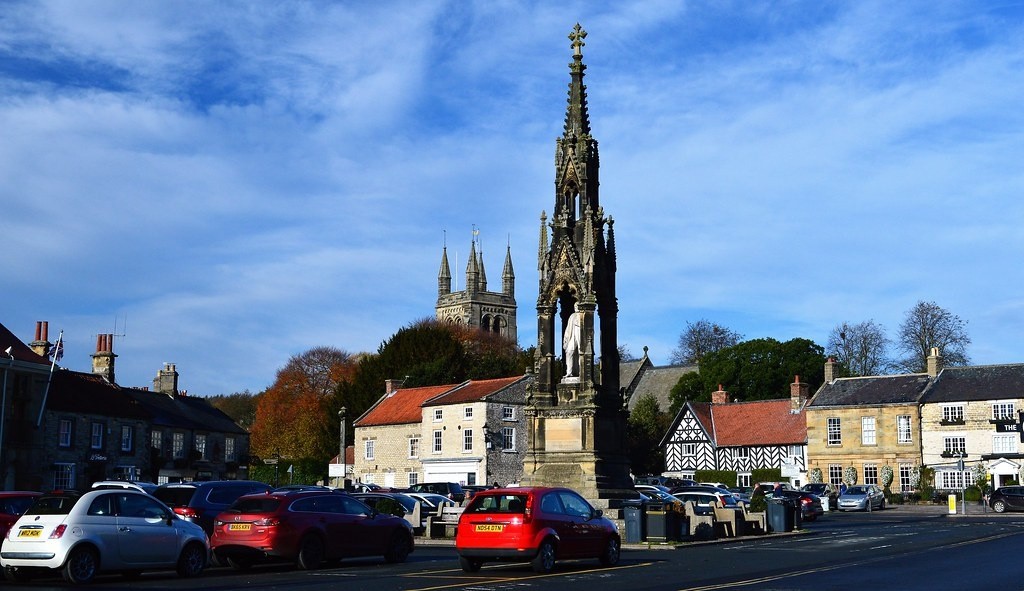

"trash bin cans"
[645,501,681,542]
[622,500,647,543]
[763,496,795,532]
[788,496,802,530]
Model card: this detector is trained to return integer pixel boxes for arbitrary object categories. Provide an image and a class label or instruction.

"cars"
[208,489,416,570]
[146,479,274,537]
[990,486,1024,513]
[275,478,502,536]
[457,485,622,573]
[837,484,886,512]
[1,490,40,542]
[0,488,213,584]
[630,482,840,522]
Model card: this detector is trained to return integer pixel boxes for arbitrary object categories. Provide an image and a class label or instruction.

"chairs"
[543,499,556,511]
[507,499,521,513]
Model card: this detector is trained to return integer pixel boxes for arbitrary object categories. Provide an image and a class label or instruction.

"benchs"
[426,501,486,539]
[685,500,764,538]
[403,501,421,529]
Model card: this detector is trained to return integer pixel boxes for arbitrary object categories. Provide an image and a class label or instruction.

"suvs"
[90,479,158,500]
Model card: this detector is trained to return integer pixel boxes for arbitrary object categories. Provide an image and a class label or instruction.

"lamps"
[482,423,490,443]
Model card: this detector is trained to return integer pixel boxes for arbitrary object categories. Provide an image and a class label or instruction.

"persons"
[750,483,765,499]
[461,490,473,507]
[492,482,502,490]
[771,482,783,498]
[562,301,580,378]
[913,488,919,505]
[839,480,856,496]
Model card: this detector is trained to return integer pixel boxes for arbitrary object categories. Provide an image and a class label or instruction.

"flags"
[47,334,64,362]
